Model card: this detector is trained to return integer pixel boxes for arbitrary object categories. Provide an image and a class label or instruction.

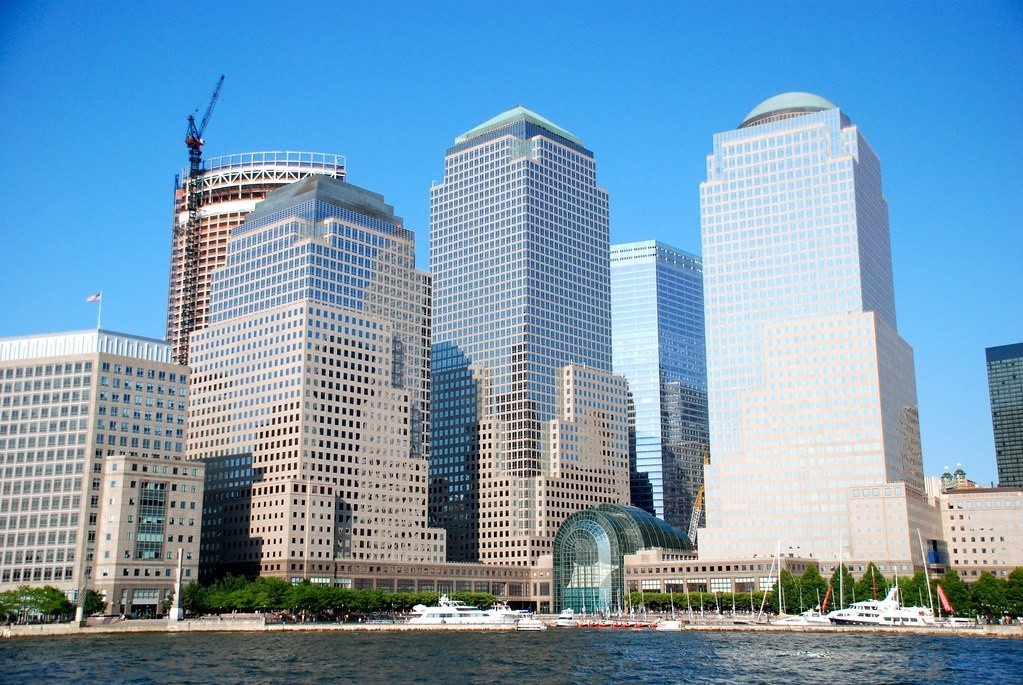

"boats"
[556,608,577,626]
[410,593,548,630]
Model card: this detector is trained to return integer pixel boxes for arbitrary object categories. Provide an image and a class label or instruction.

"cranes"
[688,444,709,553]
[180,74,226,365]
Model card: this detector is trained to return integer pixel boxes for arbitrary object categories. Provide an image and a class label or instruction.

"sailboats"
[578,526,977,632]
